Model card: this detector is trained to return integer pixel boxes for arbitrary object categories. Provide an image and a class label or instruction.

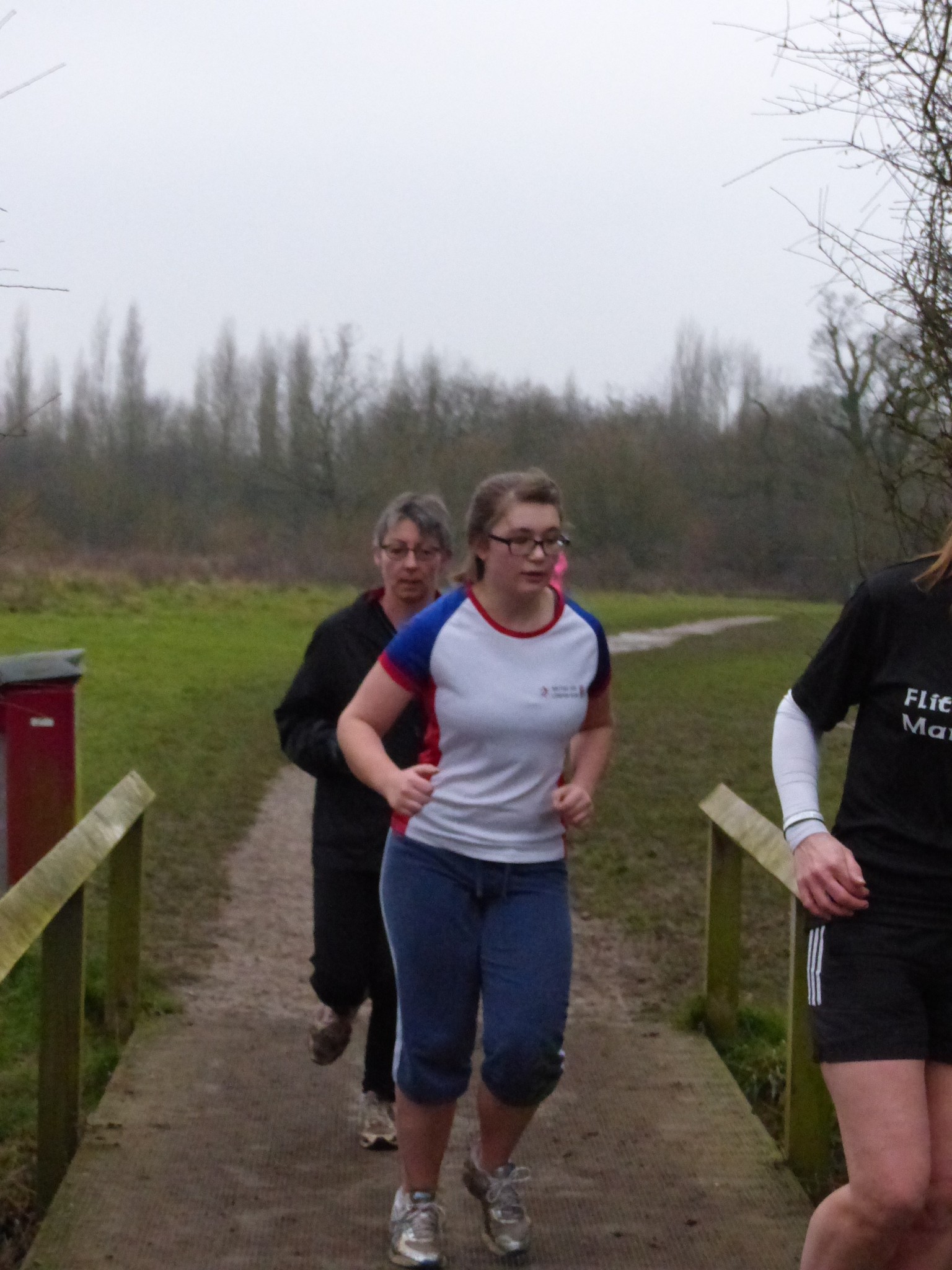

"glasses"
[488,534,571,558]
[381,541,444,562]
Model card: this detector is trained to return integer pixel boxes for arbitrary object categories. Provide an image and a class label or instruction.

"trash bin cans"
[0,649,86,900]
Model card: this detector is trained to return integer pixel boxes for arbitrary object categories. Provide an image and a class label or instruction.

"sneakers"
[386,1186,448,1270]
[459,1146,532,1257]
[308,1003,352,1065]
[358,1091,399,1150]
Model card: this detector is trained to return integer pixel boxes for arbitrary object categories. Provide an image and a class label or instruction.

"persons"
[272,491,451,1148]
[771,527,952,1269]
[339,467,612,1269]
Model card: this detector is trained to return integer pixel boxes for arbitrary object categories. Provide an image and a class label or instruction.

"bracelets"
[783,811,824,839]
